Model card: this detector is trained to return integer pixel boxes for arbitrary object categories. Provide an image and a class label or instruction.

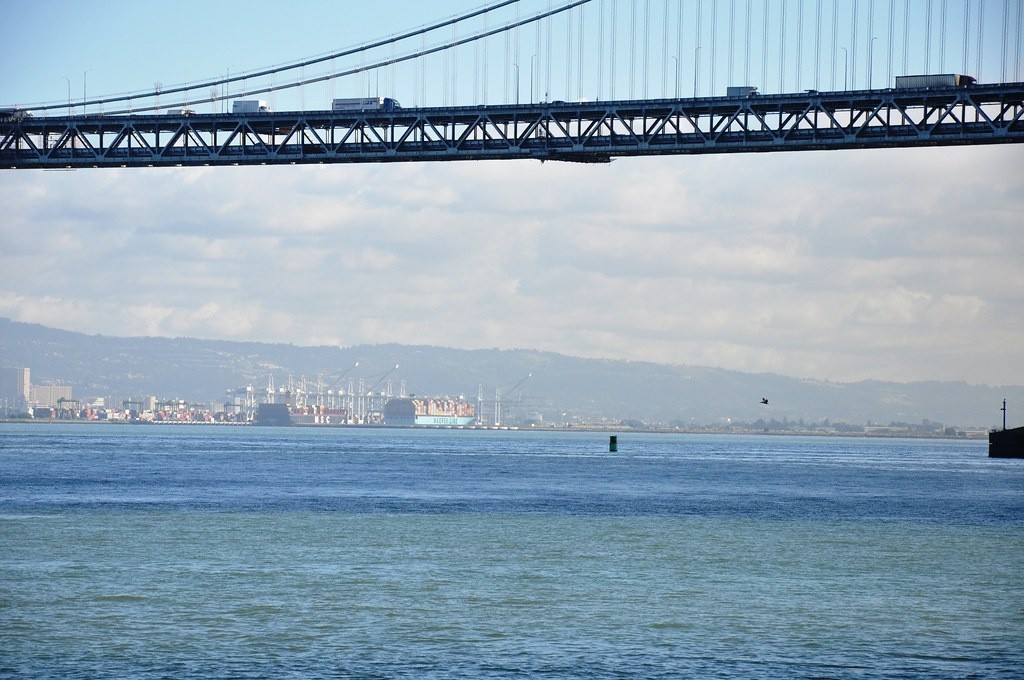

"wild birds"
[760,397,769,405]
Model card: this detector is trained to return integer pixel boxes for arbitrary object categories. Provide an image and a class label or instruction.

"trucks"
[167,109,196,114]
[896,75,976,90]
[232,101,272,113]
[727,86,760,97]
[331,97,401,111]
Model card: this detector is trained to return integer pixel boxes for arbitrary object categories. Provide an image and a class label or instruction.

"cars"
[0,106,34,124]
[551,101,565,104]
